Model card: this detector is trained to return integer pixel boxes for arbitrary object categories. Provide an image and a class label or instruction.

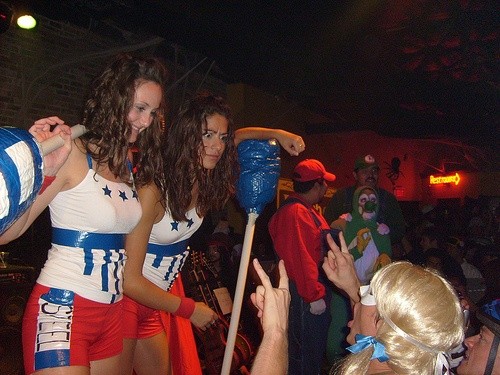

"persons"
[454,298,499,374]
[0,53,166,374]
[122,93,305,374]
[250,232,463,375]
[327,187,392,361]
[205,200,500,310]
[324,153,405,245]
[267,160,332,374]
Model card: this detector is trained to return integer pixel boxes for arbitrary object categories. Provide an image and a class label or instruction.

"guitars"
[197,251,253,366]
[190,249,247,374]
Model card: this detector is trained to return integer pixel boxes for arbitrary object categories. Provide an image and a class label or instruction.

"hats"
[293,159,336,183]
[354,154,380,170]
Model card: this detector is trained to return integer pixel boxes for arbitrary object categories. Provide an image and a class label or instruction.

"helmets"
[476,298,500,334]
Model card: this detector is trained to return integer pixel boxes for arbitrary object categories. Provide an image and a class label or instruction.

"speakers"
[0,267,38,375]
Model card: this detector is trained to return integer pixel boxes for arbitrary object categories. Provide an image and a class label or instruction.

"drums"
[231,243,277,286]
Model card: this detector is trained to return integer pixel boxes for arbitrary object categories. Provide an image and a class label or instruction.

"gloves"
[310,299,326,315]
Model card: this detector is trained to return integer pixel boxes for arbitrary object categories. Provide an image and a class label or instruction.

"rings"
[300,144,303,147]
